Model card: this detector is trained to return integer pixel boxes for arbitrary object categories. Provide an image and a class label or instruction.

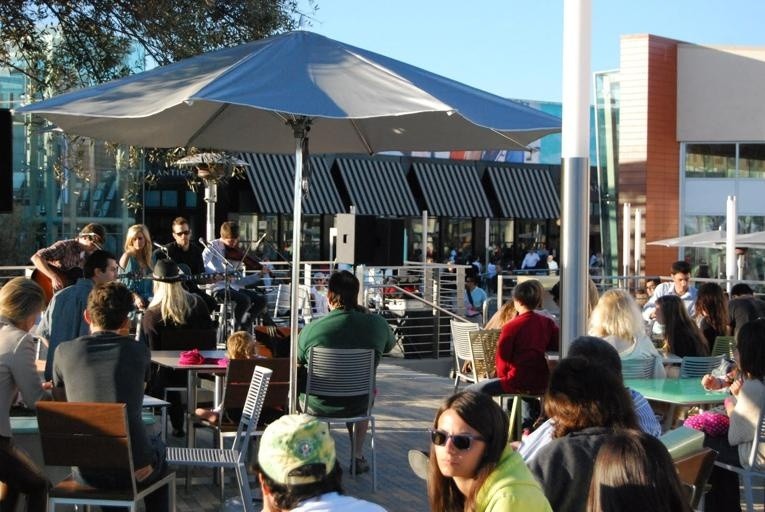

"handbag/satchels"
[685,406,729,438]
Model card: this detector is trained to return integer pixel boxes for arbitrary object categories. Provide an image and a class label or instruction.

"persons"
[1,214,396,511]
[412,242,606,327]
[425,391,554,511]
[463,245,765,512]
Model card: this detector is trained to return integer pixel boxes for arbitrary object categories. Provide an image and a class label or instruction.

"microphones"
[198,237,216,256]
[75,230,95,241]
[151,239,170,254]
[251,234,267,251]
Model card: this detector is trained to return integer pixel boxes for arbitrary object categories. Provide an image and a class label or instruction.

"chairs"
[166,366,272,512]
[254,325,290,358]
[148,313,210,401]
[184,357,291,502]
[35,401,177,512]
[296,346,377,493]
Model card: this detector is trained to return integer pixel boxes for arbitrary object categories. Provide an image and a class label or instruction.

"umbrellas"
[10,11,561,417]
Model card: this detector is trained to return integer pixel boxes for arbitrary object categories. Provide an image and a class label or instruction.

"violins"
[225,245,275,278]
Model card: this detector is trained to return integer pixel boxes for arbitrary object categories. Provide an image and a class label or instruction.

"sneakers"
[350,457,369,474]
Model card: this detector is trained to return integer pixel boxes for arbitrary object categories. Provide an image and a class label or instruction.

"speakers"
[376,216,404,267]
[335,213,376,268]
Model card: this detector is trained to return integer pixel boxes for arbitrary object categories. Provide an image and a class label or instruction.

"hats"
[259,414,336,486]
[149,260,191,282]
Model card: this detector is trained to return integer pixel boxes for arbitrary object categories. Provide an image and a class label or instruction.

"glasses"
[429,427,482,450]
[172,228,190,236]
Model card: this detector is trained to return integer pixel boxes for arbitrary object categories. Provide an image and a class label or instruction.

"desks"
[10,359,169,443]
[151,350,229,496]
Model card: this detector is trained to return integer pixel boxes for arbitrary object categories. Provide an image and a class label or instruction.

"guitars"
[31,264,143,307]
[178,262,243,292]
